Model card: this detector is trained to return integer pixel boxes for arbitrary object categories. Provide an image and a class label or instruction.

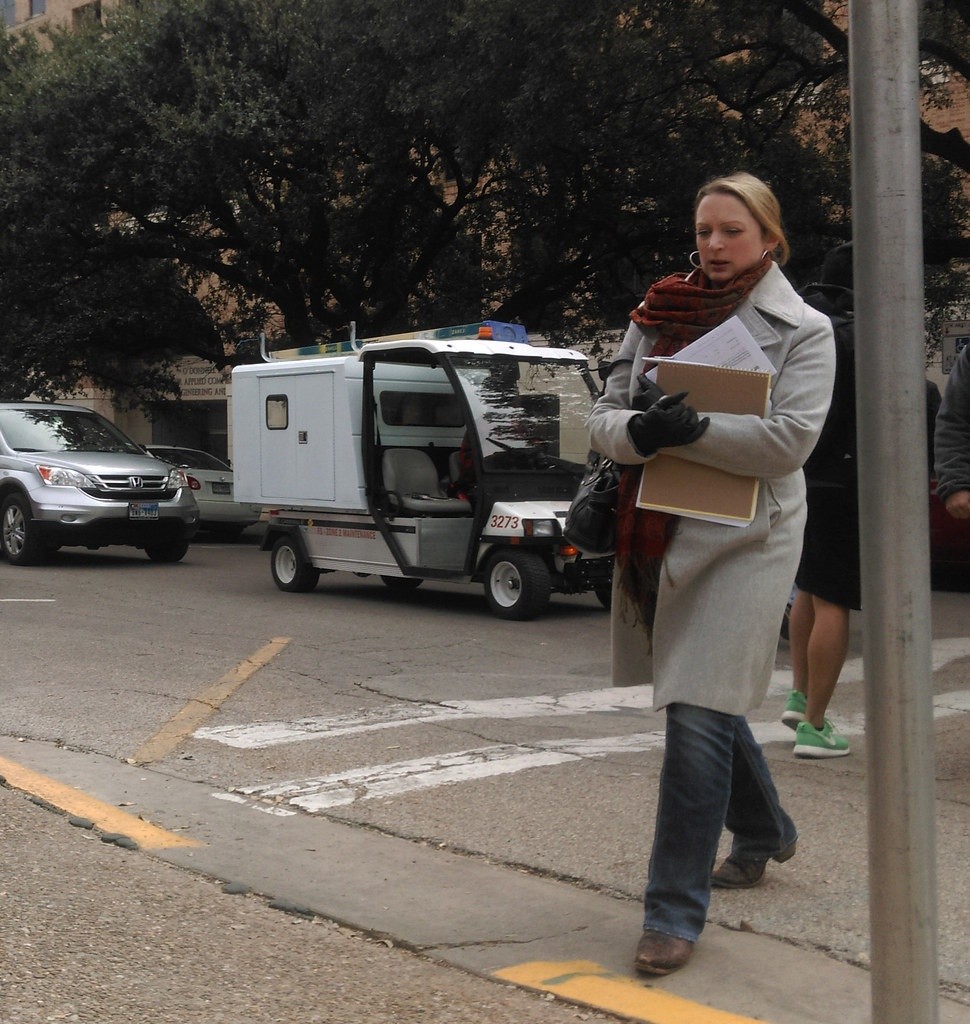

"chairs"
[449,452,460,484]
[382,449,471,515]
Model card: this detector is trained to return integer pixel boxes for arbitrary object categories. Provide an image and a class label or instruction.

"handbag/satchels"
[562,450,623,557]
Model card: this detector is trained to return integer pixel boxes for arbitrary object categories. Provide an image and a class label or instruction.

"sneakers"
[793,717,850,759]
[780,689,807,731]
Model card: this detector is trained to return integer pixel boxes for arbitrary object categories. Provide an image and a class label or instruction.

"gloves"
[627,390,710,457]
[630,373,667,412]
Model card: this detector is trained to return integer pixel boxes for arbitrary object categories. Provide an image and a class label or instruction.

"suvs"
[0,400,202,564]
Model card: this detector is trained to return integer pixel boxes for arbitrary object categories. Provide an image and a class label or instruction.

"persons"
[934,337,970,520]
[778,235,942,757]
[581,172,840,975]
[459,374,548,491]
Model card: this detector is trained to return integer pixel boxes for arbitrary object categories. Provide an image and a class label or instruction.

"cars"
[126,444,262,540]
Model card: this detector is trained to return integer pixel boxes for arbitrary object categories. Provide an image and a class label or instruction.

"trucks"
[232,320,618,621]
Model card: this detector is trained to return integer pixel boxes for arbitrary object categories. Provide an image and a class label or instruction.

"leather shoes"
[633,929,694,974]
[711,839,797,889]
